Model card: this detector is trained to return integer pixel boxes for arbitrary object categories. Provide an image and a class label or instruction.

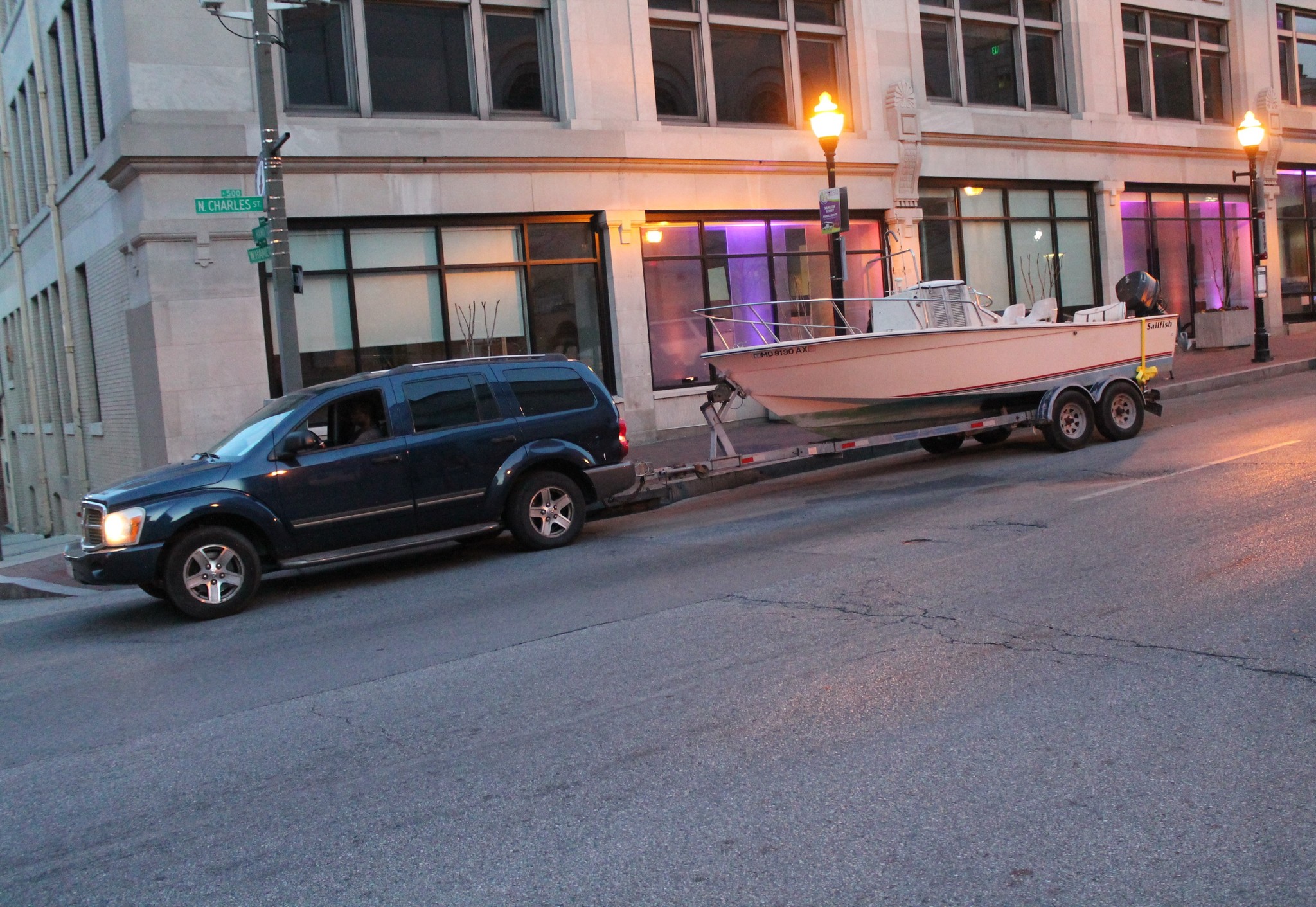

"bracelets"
[319,442,324,446]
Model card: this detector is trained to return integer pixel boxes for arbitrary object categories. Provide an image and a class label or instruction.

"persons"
[318,404,383,450]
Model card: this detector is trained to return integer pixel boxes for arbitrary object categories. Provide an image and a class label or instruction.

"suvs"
[61,351,638,622]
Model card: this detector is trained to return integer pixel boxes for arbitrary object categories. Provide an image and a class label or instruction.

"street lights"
[808,89,850,336]
[1236,110,1274,363]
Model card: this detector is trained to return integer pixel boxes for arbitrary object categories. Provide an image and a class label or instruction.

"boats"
[689,246,1189,441]
